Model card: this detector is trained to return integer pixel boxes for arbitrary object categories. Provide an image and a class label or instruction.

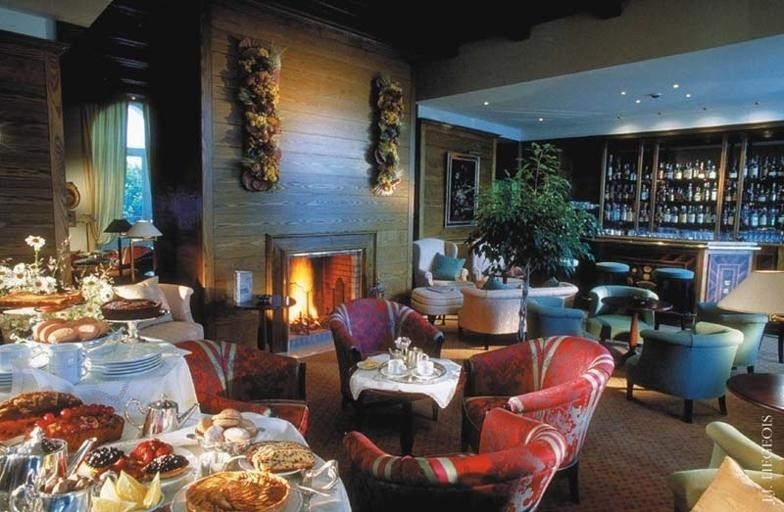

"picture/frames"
[443,151,482,231]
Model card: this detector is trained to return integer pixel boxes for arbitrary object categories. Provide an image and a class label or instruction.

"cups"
[415,359,435,375]
[232,269,254,303]
[388,359,405,376]
[48,345,88,384]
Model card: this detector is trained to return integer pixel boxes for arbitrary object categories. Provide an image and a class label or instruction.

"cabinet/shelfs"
[597,137,657,234]
[737,136,784,242]
[653,138,744,240]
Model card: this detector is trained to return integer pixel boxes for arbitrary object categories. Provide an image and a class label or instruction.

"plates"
[410,367,440,379]
[186,427,266,452]
[88,343,163,378]
[170,475,303,511]
[356,360,379,370]
[143,492,166,511]
[238,440,302,476]
[87,442,198,486]
[380,367,409,377]
[0,343,49,389]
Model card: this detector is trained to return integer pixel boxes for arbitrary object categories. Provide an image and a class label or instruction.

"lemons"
[90,470,161,512]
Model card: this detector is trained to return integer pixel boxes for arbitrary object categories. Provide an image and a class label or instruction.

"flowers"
[232,33,288,193]
[370,70,407,198]
[1,232,114,321]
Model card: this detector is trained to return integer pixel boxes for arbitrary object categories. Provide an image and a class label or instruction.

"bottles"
[606,153,637,181]
[604,181,636,202]
[721,158,738,231]
[740,205,784,230]
[656,206,717,228]
[604,203,634,229]
[639,163,651,227]
[744,153,784,179]
[657,160,719,182]
[742,179,784,203]
[654,182,718,205]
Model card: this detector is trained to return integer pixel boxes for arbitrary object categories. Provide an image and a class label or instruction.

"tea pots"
[388,347,406,360]
[7,471,97,512]
[0,426,97,491]
[122,393,199,437]
[402,346,429,367]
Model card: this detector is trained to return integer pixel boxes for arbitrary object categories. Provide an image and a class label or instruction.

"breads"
[140,454,190,480]
[128,437,175,471]
[0,390,83,446]
[101,298,161,318]
[32,316,109,345]
[186,471,289,512]
[245,440,315,472]
[197,407,257,442]
[78,446,129,478]
[24,404,124,452]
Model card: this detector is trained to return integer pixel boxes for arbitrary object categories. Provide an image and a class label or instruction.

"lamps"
[122,221,163,280]
[102,217,134,275]
[77,212,96,253]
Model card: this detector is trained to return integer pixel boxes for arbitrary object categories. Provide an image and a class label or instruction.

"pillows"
[113,274,177,333]
